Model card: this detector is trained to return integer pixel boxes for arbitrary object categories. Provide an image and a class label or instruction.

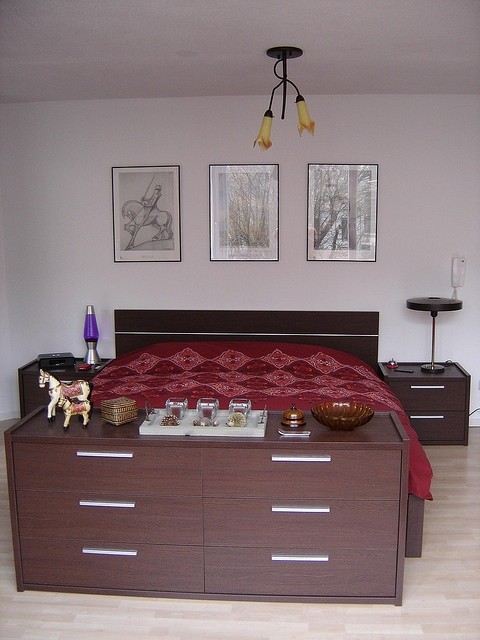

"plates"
[311,402,373,432]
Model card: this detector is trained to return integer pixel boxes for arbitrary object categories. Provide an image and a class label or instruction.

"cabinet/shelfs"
[3,405,410,607]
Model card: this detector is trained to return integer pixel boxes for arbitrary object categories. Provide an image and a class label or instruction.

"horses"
[58,394,93,430]
[122,199,174,251]
[39,369,93,423]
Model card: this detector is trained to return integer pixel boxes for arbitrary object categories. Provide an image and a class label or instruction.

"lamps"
[407,296,462,372]
[251,45,314,150]
[82,305,102,365]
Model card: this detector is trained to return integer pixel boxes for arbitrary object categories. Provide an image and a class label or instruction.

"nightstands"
[378,360,471,446]
[18,356,115,420]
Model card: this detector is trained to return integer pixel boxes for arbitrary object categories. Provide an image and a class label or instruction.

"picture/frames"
[111,165,181,262]
[209,164,279,261]
[307,163,378,262]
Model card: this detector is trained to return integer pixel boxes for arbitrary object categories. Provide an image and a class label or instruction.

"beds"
[87,310,434,559]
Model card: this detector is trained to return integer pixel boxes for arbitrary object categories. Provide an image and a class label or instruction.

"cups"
[165,397,188,420]
[197,398,219,420]
[229,399,251,419]
